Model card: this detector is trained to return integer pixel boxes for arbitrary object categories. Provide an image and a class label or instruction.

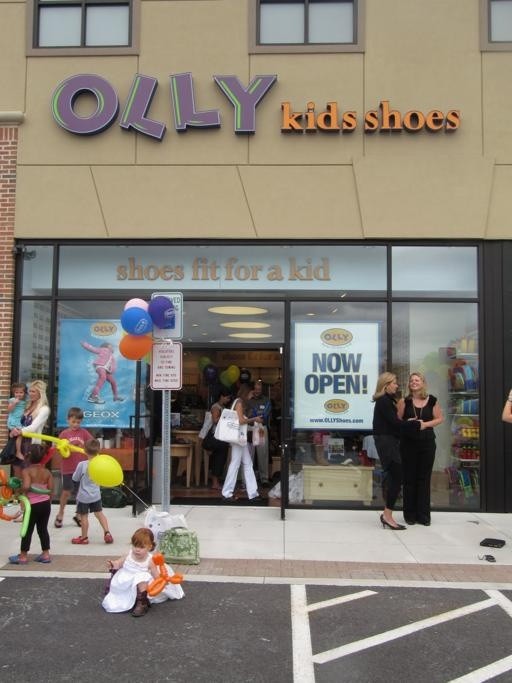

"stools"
[271,455,285,479]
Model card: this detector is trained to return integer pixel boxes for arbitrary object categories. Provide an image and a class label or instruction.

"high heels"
[380,514,407,530]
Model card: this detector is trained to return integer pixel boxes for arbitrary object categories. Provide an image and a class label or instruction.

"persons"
[54,407,94,528]
[72,439,114,544]
[370,373,425,530]
[101,528,185,617]
[8,444,54,564]
[202,377,272,502]
[501,389,512,423]
[80,339,124,404]
[396,372,443,526]
[7,383,28,461]
[9,379,51,523]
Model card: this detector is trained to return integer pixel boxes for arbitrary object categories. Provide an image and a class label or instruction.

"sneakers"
[409,520,431,526]
[113,397,124,402]
[211,481,268,504]
[87,394,105,404]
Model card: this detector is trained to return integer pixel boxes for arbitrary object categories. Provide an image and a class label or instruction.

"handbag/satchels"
[156,526,201,566]
[198,411,229,452]
[213,398,248,447]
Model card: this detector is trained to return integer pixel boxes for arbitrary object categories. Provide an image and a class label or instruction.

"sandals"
[34,554,51,563]
[55,515,62,528]
[104,531,113,544]
[8,554,28,565]
[73,516,82,527]
[72,535,89,545]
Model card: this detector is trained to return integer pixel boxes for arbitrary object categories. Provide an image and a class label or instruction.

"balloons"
[21,431,88,458]
[0,468,53,537]
[87,454,125,488]
[147,551,183,597]
[198,356,251,388]
[119,296,175,361]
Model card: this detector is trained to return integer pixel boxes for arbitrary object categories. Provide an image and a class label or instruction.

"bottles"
[447,395,480,415]
[446,334,477,356]
[450,426,481,460]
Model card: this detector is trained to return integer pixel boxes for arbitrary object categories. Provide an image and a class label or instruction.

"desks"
[173,425,216,486]
[169,442,197,491]
[55,446,145,492]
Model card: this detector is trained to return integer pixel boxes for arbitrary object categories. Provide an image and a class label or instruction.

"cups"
[92,428,135,450]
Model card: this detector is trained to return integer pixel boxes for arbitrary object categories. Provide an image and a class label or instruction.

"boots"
[131,590,151,618]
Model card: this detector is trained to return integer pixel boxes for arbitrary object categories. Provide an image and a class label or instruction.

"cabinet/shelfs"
[445,350,480,464]
[300,462,375,508]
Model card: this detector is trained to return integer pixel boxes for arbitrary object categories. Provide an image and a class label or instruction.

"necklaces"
[412,397,424,420]
